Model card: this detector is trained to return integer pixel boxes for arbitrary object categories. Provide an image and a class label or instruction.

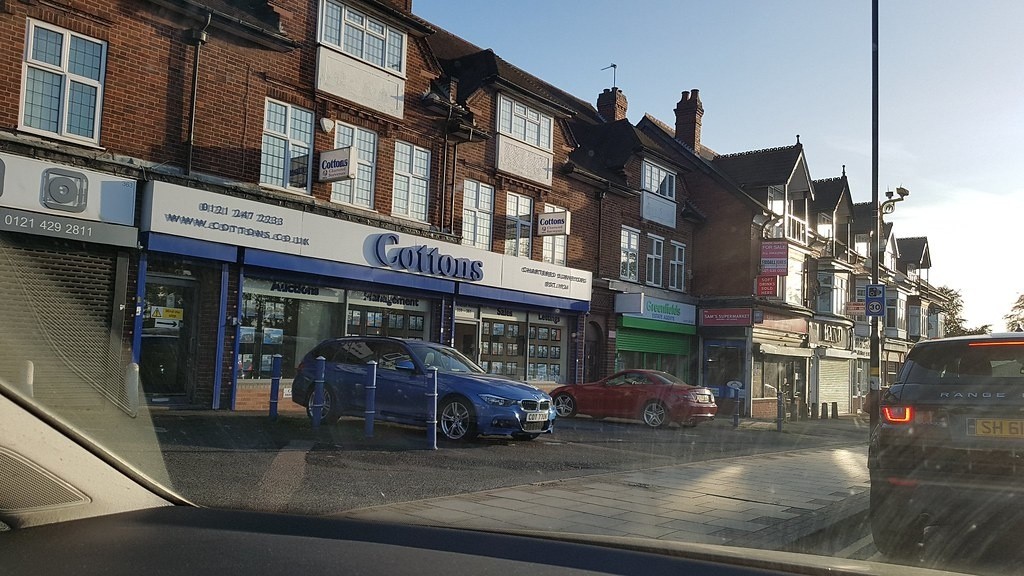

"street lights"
[870,0,908,463]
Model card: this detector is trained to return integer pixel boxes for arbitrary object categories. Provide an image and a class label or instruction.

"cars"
[548,369,718,429]
[125,328,180,392]
[868,332,1024,558]
[239,332,277,374]
[290,336,556,444]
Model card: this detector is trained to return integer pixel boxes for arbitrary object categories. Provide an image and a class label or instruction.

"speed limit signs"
[868,300,883,314]
